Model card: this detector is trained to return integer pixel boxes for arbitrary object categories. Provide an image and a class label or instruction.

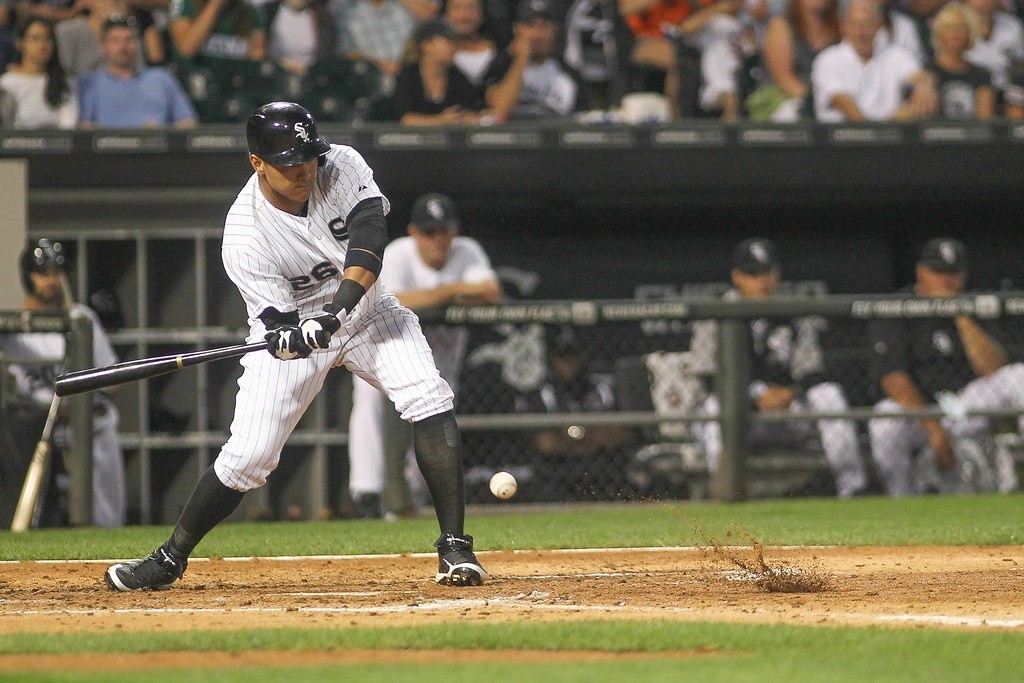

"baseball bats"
[10,332,75,532]
[54,328,331,397]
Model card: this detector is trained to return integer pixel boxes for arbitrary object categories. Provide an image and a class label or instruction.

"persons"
[4,236,126,526]
[867,239,1010,499]
[105,104,488,593]
[0,0,422,133]
[512,336,638,503]
[392,0,1024,127]
[697,256,890,498]
[348,190,505,522]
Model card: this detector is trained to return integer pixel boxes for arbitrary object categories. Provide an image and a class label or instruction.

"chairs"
[616,343,1024,494]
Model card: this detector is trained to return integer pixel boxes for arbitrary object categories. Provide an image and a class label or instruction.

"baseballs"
[491,471,518,499]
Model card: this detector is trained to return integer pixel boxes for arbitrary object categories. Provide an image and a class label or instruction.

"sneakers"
[433,532,488,587]
[103,545,188,592]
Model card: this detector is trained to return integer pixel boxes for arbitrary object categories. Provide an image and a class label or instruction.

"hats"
[734,238,777,276]
[413,194,458,234]
[924,238,967,270]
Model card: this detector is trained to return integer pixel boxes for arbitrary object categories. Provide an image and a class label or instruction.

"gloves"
[299,301,347,349]
[264,324,308,361]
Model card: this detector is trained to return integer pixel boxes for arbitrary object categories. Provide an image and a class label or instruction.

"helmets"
[246,101,333,167]
[21,239,68,289]
